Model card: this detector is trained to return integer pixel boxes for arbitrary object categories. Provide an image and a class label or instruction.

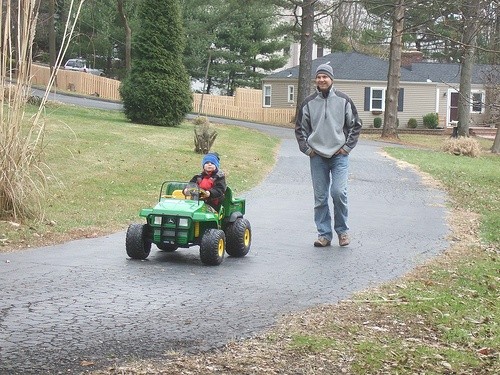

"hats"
[315,61,334,81]
[202,153,220,170]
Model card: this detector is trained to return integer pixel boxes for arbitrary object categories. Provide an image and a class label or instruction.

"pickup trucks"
[125,180,252,266]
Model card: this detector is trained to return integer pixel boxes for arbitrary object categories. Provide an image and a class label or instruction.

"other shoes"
[314,237,331,246]
[338,232,350,245]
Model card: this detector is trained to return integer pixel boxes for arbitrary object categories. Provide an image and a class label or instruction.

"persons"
[294,61,363,247]
[189,152,226,214]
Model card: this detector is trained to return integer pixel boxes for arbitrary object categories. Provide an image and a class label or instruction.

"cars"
[64,57,106,77]
